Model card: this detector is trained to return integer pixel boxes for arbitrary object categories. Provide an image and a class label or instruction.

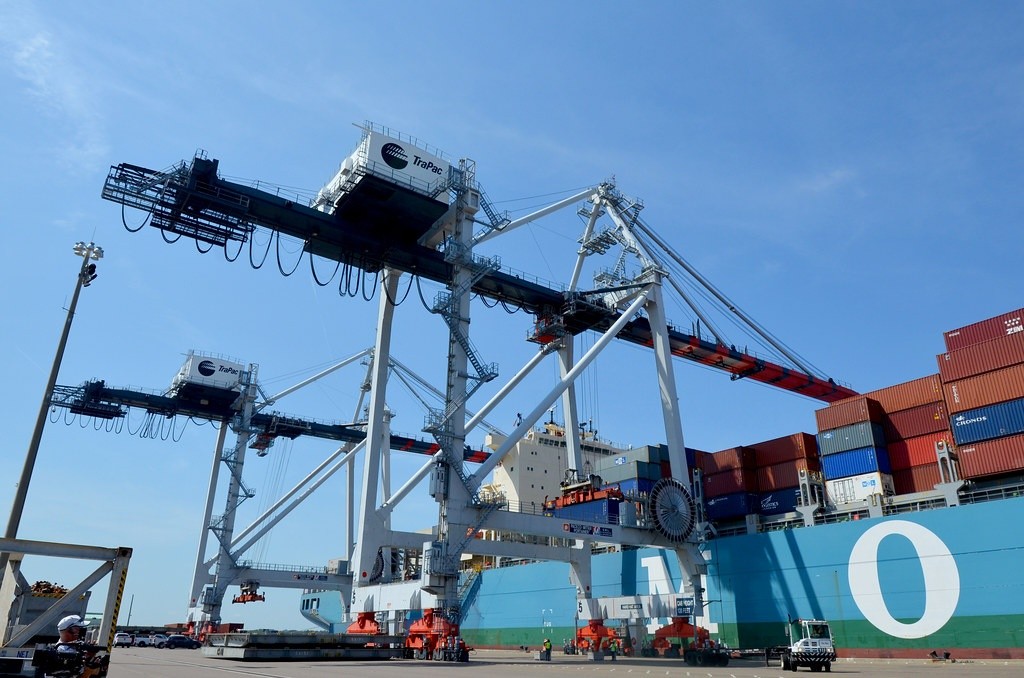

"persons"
[55,615,101,678]
[543,638,552,661]
[611,639,617,661]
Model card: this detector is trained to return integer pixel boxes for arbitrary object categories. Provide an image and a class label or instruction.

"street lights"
[6,241,104,539]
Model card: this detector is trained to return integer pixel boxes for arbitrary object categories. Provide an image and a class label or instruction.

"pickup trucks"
[112,631,166,648]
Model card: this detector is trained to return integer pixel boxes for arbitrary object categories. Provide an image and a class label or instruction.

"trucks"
[763,618,837,673]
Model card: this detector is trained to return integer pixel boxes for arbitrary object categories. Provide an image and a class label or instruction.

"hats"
[58,614,91,631]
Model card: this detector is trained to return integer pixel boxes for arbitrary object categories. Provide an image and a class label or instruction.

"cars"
[165,633,202,651]
